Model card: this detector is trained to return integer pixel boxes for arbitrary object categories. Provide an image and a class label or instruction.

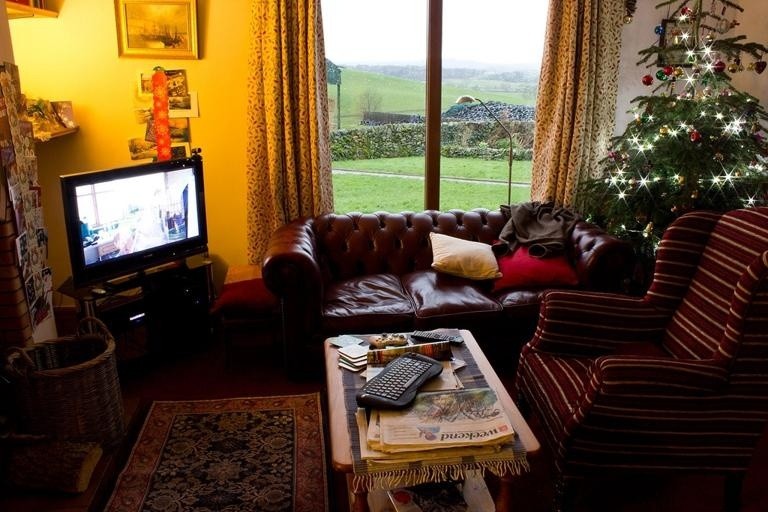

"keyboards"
[355,351,443,410]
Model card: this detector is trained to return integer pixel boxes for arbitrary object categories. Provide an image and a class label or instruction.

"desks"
[57,251,217,383]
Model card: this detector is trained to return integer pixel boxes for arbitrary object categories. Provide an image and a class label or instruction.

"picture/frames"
[114,0,199,59]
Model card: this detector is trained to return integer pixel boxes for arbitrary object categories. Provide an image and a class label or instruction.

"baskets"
[4,316,128,449]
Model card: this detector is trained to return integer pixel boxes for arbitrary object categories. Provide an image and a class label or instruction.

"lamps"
[456,95,512,206]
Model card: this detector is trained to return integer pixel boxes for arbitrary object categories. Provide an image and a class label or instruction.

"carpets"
[89,390,339,511]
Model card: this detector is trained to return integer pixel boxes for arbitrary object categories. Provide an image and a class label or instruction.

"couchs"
[262,205,636,385]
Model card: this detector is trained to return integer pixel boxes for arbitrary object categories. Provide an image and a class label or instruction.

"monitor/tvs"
[57,156,208,287]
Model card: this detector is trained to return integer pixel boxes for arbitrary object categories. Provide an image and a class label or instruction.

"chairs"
[515,206,768,512]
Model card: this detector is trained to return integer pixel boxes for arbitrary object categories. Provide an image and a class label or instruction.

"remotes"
[412,330,467,345]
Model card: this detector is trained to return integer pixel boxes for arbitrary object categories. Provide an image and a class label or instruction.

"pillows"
[430,232,581,293]
